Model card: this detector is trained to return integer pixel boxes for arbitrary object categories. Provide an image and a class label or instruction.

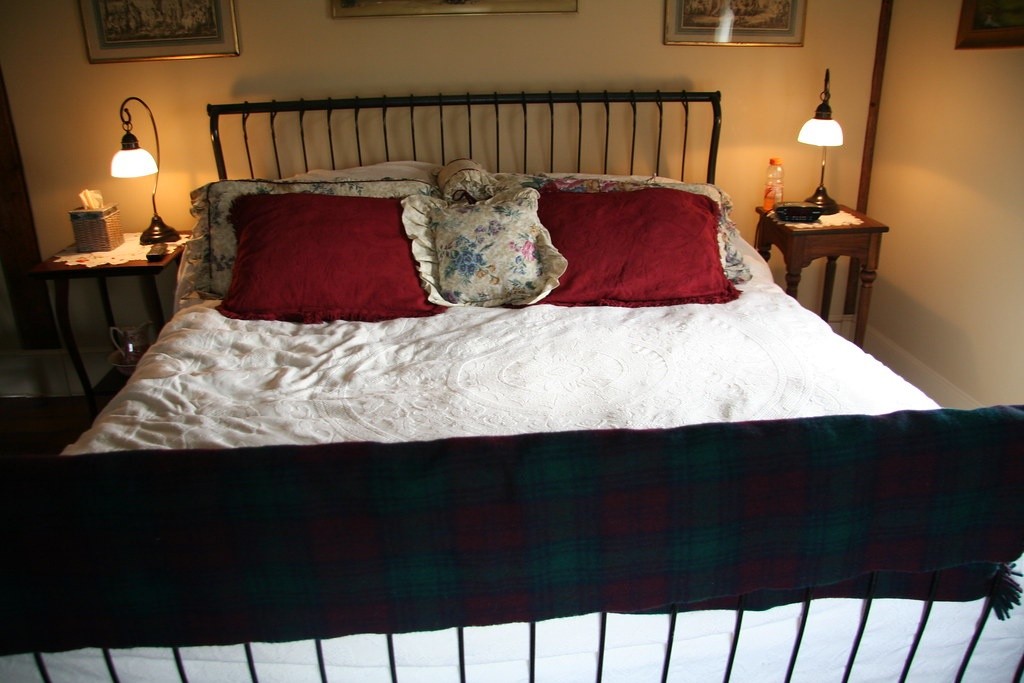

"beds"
[0,90,1023,683]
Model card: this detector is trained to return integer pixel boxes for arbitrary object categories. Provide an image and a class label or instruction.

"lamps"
[109,96,180,246]
[797,68,843,215]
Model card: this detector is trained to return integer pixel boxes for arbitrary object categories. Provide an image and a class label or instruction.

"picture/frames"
[76,0,241,64]
[662,0,808,48]
[954,0,1024,51]
[330,0,579,21]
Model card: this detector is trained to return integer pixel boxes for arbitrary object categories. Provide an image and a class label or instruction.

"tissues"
[67,189,125,253]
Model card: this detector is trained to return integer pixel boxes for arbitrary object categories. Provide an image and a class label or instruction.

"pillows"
[480,168,754,285]
[214,192,449,325]
[400,187,569,308]
[181,169,443,302]
[537,188,743,308]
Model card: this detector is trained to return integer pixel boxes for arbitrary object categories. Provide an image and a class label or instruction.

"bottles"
[763,157,785,210]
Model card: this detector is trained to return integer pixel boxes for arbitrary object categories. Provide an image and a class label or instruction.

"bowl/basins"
[107,340,155,377]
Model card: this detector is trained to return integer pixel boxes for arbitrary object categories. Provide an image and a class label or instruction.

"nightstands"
[754,202,889,349]
[29,228,192,426]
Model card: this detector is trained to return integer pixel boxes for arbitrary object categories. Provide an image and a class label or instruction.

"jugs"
[110,320,154,364]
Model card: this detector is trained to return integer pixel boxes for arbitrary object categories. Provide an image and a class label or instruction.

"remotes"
[146,243,168,262]
